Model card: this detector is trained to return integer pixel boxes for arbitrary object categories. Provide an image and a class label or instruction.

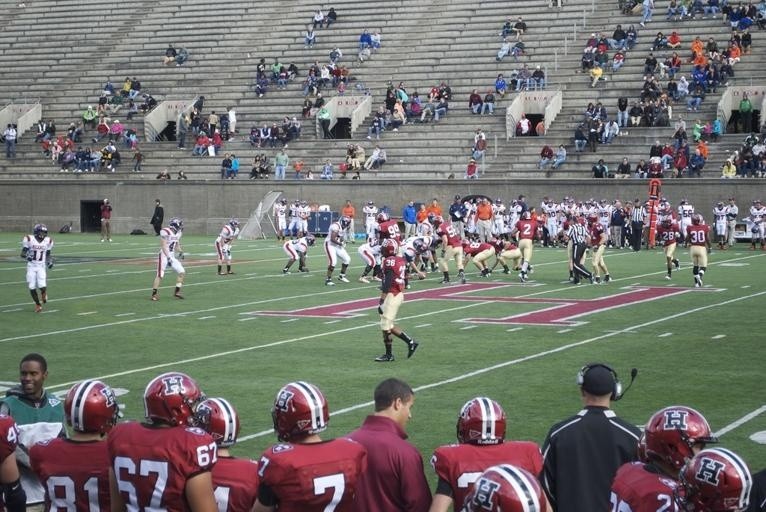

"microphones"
[620,368,638,398]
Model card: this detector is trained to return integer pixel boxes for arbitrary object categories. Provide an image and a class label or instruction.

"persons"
[684,214,711,286]
[509,69,518,90]
[462,239,496,277]
[358,242,382,284]
[496,40,510,62]
[631,199,647,252]
[492,72,508,97]
[722,130,766,180]
[120,77,131,98]
[498,18,514,41]
[468,90,483,115]
[357,45,370,67]
[512,17,527,42]
[405,233,443,268]
[130,77,141,99]
[147,96,157,110]
[288,200,300,240]
[363,201,378,243]
[0,414,27,512]
[20,223,53,313]
[229,154,238,179]
[399,236,426,289]
[298,200,310,237]
[366,82,452,141]
[721,0,766,86]
[712,201,728,248]
[359,30,371,50]
[325,7,336,27]
[150,199,163,237]
[344,144,387,172]
[129,132,137,150]
[30,380,120,512]
[739,93,752,134]
[321,159,333,180]
[431,397,553,512]
[344,379,433,512]
[378,212,401,246]
[726,198,738,248]
[157,169,170,179]
[677,200,695,248]
[416,204,430,234]
[427,199,442,219]
[250,115,301,149]
[479,89,496,116]
[255,56,298,98]
[515,65,531,91]
[540,196,631,285]
[491,235,534,275]
[590,161,605,179]
[302,60,349,97]
[1,354,65,512]
[519,195,528,215]
[464,198,473,236]
[214,219,239,274]
[475,198,493,242]
[610,405,719,512]
[655,219,683,281]
[293,160,304,179]
[474,128,487,144]
[107,372,217,511]
[250,152,261,180]
[532,65,545,90]
[599,159,609,178]
[537,146,553,169]
[472,136,489,162]
[126,99,138,121]
[516,113,532,137]
[33,92,123,175]
[449,195,466,240]
[581,0,630,88]
[274,198,287,240]
[164,44,176,63]
[342,200,356,243]
[3,124,17,158]
[630,0,721,127]
[492,198,506,235]
[125,128,137,145]
[177,46,188,65]
[403,199,418,239]
[195,396,260,512]
[512,39,525,61]
[466,158,477,181]
[303,97,313,120]
[304,25,315,46]
[432,215,466,284]
[283,235,315,275]
[313,9,325,29]
[575,95,619,152]
[538,364,642,511]
[219,153,231,178]
[648,126,721,181]
[305,170,314,180]
[101,81,115,98]
[178,96,237,156]
[141,95,148,110]
[535,118,546,136]
[374,238,419,363]
[100,198,113,243]
[420,211,439,271]
[370,30,381,49]
[324,213,353,285]
[315,93,325,108]
[259,381,366,512]
[750,200,766,250]
[467,197,480,241]
[509,199,523,241]
[151,217,186,302]
[260,153,271,179]
[130,150,145,172]
[553,143,567,169]
[274,150,288,181]
[614,157,632,180]
[505,212,541,283]
[634,160,649,179]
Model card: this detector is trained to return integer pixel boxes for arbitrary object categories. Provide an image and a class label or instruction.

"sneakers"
[518,273,524,283]
[530,265,535,274]
[602,277,611,282]
[723,244,726,249]
[460,272,466,284]
[592,279,601,285]
[590,272,596,283]
[751,245,755,251]
[696,276,703,285]
[228,271,235,274]
[217,272,226,275]
[674,259,680,270]
[42,293,48,303]
[694,281,700,288]
[716,244,721,249]
[502,269,510,274]
[151,293,160,300]
[375,354,395,362]
[481,270,488,278]
[175,293,185,299]
[574,278,581,284]
[325,280,335,286]
[298,268,307,273]
[372,276,382,281]
[101,239,105,243]
[440,279,451,284]
[109,239,113,242]
[35,304,42,313]
[408,340,419,357]
[338,276,350,283]
[489,269,492,276]
[359,277,370,283]
[284,268,288,274]
[569,276,574,282]
[761,245,766,249]
[523,272,528,279]
[666,275,673,280]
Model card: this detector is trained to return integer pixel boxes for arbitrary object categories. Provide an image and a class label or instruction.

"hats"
[456,195,461,200]
[584,366,613,395]
[519,193,525,201]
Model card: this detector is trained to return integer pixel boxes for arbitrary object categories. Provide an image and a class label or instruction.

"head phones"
[576,362,638,403]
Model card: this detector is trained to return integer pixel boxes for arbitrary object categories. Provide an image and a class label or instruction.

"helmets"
[306,234,316,247]
[192,395,241,449]
[463,464,547,512]
[340,215,351,228]
[462,239,470,247]
[490,239,497,245]
[33,222,48,238]
[522,211,531,220]
[170,216,183,232]
[229,218,241,229]
[662,215,672,228]
[381,238,400,258]
[413,239,424,251]
[587,214,597,223]
[544,195,609,209]
[272,381,329,440]
[691,215,700,225]
[142,372,200,424]
[280,198,287,205]
[295,199,308,208]
[377,213,390,223]
[639,405,719,474]
[433,216,443,225]
[456,395,508,446]
[368,201,374,208]
[64,379,120,436]
